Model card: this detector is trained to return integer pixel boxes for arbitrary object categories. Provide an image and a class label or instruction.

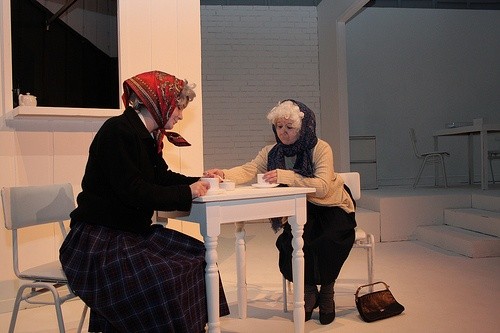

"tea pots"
[19,92,37,107]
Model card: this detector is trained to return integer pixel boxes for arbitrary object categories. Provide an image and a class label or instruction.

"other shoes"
[305,291,336,325]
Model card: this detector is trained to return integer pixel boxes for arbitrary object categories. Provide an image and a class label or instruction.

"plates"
[251,183,280,187]
[205,189,226,194]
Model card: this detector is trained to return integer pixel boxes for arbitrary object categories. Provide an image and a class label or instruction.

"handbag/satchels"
[354,281,404,323]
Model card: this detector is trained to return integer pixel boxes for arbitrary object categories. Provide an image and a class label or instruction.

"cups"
[198,178,220,190]
[220,183,236,190]
[257,174,270,185]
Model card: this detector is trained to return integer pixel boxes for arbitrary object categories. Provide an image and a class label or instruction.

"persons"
[202,99,357,325]
[59,70,231,333]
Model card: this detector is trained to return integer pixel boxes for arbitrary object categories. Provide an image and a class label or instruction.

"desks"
[432,125,500,189]
[150,187,316,333]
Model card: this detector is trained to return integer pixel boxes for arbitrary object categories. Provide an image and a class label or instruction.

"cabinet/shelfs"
[350,136,377,190]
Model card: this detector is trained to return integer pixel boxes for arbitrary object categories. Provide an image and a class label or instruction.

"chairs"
[1,183,88,333]
[488,151,500,184]
[410,127,451,189]
[282,172,375,312]
